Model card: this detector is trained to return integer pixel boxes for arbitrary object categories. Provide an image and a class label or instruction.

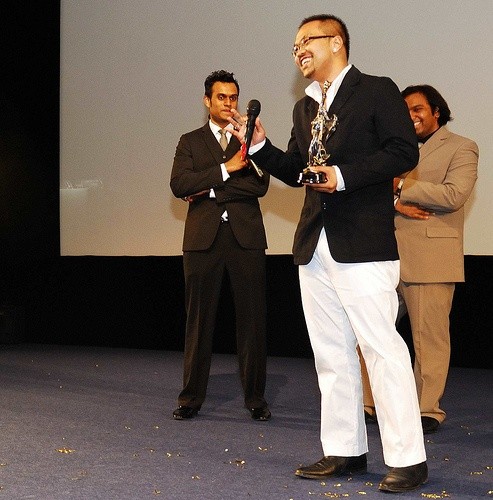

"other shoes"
[173,406,199,420]
[421,417,440,435]
[248,405,272,421]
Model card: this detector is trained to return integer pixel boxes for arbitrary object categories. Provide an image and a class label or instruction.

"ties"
[217,129,229,151]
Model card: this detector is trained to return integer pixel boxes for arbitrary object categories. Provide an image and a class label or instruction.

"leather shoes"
[295,454,369,480]
[378,462,429,494]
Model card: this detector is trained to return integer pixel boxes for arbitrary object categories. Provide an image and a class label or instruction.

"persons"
[171,67,274,423]
[219,15,428,492]
[363,86,478,427]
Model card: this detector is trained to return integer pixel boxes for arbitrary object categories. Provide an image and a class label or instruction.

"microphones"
[242,100,261,161]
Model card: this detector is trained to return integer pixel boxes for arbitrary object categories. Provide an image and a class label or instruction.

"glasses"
[291,35,336,57]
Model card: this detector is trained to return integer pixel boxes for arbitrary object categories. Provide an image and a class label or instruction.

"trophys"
[296,76,338,185]
